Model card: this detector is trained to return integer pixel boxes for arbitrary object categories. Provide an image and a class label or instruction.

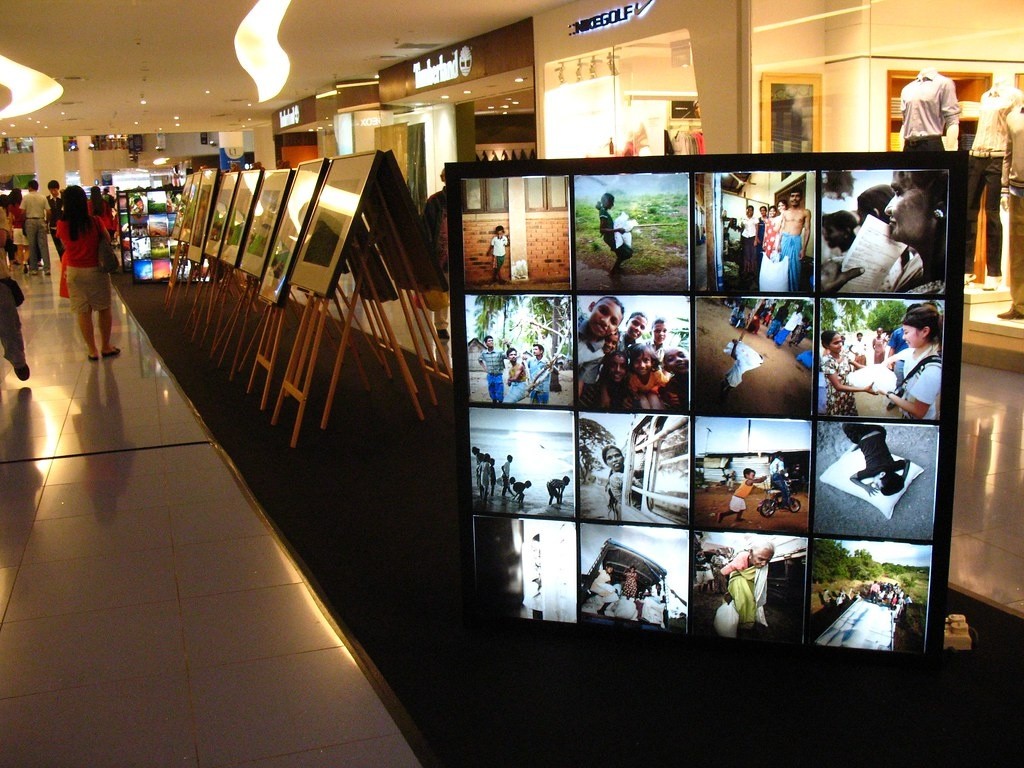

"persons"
[823,581,913,621]
[738,188,811,292]
[964,78,1022,290]
[3,137,11,154]
[66,137,75,150]
[720,297,809,396]
[592,563,666,616]
[601,445,645,510]
[0,206,30,381]
[134,198,144,210]
[472,446,570,507]
[0,179,115,275]
[55,185,121,360]
[485,225,510,282]
[820,302,942,417]
[997,104,1024,320]
[718,450,792,523]
[899,67,959,150]
[477,335,552,405]
[695,541,775,631]
[843,423,911,498]
[15,137,23,153]
[577,296,689,410]
[521,533,543,611]
[595,193,634,278]
[423,169,450,339]
[819,169,946,294]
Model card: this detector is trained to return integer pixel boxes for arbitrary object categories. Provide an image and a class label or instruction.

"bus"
[615,415,689,525]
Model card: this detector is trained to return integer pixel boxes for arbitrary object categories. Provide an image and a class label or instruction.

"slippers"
[88,354,98,360]
[102,347,120,356]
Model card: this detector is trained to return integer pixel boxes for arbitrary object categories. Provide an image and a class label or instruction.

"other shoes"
[29,270,37,275]
[23,261,28,274]
[437,328,450,340]
[717,513,723,524]
[14,364,29,381]
[997,305,1024,319]
[735,518,746,522]
[45,270,51,275]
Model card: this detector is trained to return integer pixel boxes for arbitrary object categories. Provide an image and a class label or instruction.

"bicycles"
[757,479,801,517]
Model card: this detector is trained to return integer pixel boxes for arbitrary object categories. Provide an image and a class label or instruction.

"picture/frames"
[204,171,239,259]
[287,150,386,298]
[257,158,328,308]
[217,169,263,269]
[344,215,399,304]
[236,168,292,281]
[171,174,194,240]
[179,171,202,242]
[761,72,822,150]
[186,167,217,264]
[362,150,448,292]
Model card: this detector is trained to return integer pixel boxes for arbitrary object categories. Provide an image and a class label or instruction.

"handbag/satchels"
[0,279,25,307]
[415,289,449,312]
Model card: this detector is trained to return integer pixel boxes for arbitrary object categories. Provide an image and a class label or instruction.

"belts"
[969,150,1005,158]
[26,217,44,219]
[905,136,940,146]
[1009,180,1024,187]
